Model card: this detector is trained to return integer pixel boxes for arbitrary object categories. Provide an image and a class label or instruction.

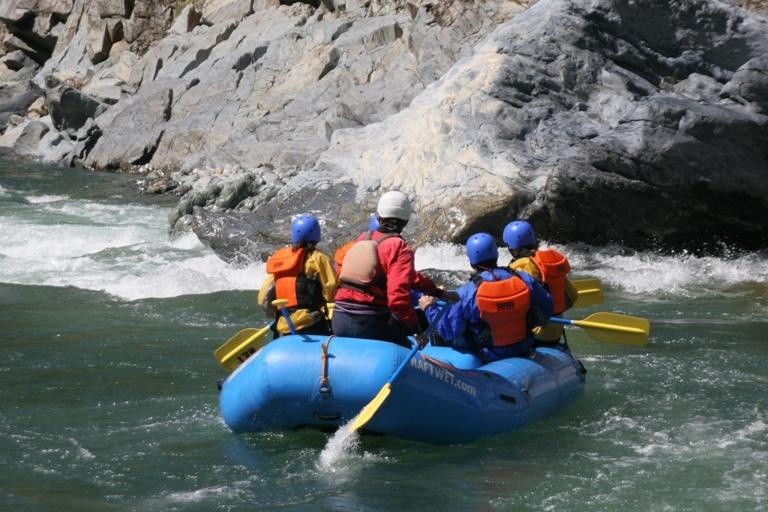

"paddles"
[550,313,650,347]
[569,280,604,308]
[344,298,455,433]
[213,318,278,370]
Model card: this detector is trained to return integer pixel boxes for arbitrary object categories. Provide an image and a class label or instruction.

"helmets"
[503,221,536,250]
[291,216,321,243]
[466,233,498,268]
[368,191,409,231]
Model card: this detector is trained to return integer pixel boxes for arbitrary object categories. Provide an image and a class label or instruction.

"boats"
[218,288,589,445]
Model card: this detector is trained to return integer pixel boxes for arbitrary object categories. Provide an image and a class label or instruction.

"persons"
[256,213,340,336]
[331,191,462,350]
[418,219,578,362]
